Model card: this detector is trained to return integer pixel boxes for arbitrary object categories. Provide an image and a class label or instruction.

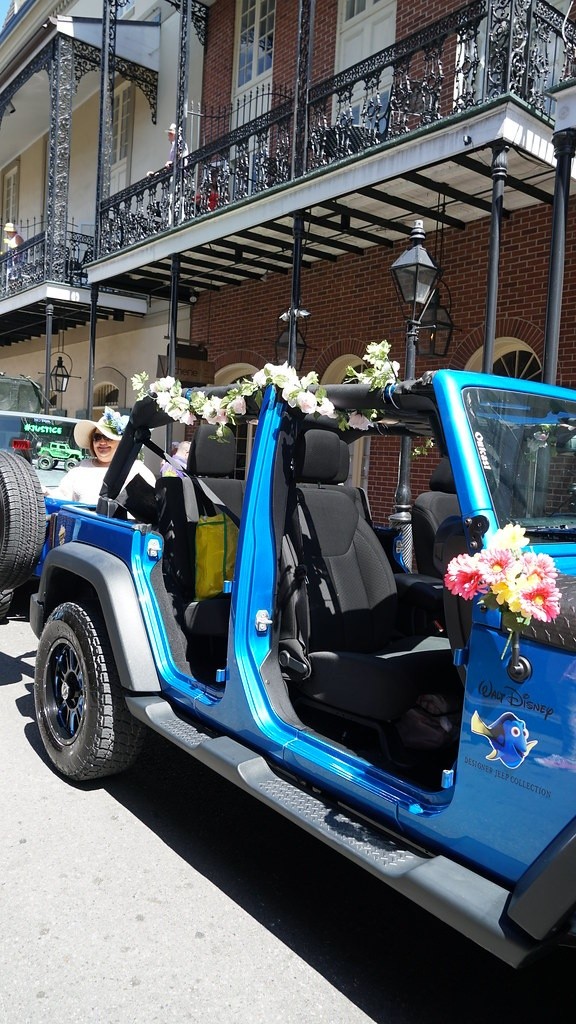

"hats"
[165,123,182,135]
[3,223,16,232]
[74,406,130,449]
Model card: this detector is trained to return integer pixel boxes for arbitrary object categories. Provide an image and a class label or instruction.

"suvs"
[30,369,576,996]
[0,411,90,625]
[36,441,83,472]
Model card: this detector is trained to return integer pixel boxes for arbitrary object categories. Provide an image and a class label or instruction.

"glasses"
[93,433,113,441]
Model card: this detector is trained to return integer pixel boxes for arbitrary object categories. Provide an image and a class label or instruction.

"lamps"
[50,305,71,393]
[414,187,461,360]
[5,102,16,113]
[273,209,314,372]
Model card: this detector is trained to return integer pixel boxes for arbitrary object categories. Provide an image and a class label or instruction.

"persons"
[147,122,189,227]
[159,440,191,477]
[46,408,156,520]
[2,223,25,281]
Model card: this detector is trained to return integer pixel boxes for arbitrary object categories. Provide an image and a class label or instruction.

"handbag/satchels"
[195,512,239,601]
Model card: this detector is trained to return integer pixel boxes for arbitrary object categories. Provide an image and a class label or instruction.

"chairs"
[390,81,444,135]
[288,440,372,550]
[278,431,456,721]
[154,424,249,644]
[316,125,381,159]
[411,455,511,642]
[73,246,95,284]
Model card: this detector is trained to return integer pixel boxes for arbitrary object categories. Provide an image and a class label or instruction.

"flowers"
[129,339,576,466]
[443,524,563,658]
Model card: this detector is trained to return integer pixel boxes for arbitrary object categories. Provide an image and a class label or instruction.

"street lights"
[388,218,437,573]
